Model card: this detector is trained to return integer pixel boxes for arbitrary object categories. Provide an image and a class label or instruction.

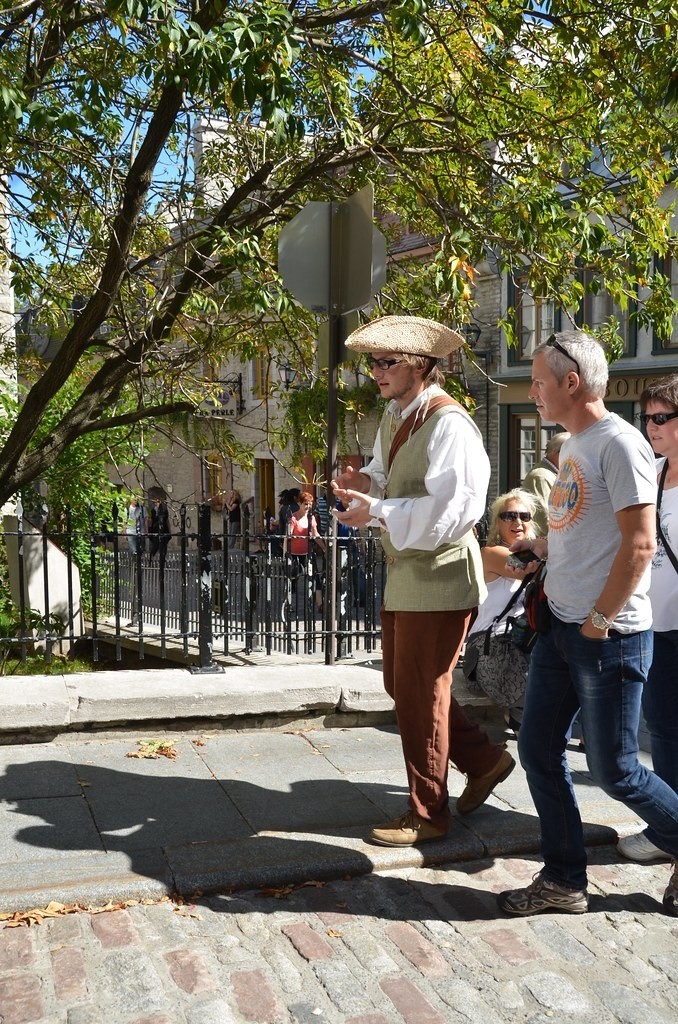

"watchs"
[586,608,612,631]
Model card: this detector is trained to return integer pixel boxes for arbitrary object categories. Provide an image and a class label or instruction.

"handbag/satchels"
[525,579,552,636]
[465,630,532,706]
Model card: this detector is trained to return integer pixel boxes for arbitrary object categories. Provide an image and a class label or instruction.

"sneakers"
[616,829,674,860]
[497,873,590,916]
[663,857,678,916]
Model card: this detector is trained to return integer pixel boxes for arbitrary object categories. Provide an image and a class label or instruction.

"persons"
[522,431,575,534]
[612,368,677,863]
[341,339,517,848]
[499,333,677,914]
[466,487,540,730]
[89,484,363,612]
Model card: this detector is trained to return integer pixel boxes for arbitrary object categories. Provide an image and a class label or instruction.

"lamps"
[457,323,493,366]
[278,359,310,392]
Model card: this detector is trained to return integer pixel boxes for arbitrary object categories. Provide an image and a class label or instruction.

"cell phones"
[503,549,541,569]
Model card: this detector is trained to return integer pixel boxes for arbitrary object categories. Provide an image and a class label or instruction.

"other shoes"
[457,748,516,814]
[369,808,450,848]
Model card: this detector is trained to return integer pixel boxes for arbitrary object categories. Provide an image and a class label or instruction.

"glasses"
[366,355,404,370]
[546,332,582,377]
[634,410,677,425]
[496,511,531,521]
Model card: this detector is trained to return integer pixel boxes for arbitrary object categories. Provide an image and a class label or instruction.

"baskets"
[344,315,467,359]
